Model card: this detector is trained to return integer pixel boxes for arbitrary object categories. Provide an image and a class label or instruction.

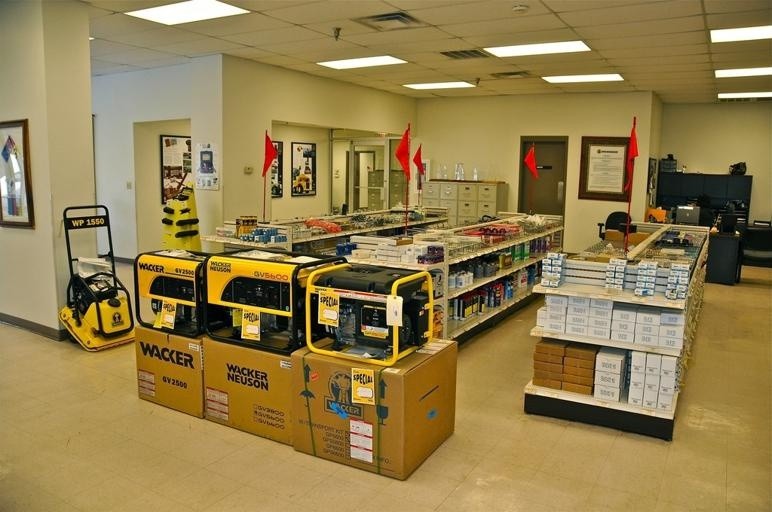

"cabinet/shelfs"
[420,180,510,227]
[201,205,450,255]
[342,210,566,347]
[523,218,713,442]
[367,169,407,210]
[655,170,755,286]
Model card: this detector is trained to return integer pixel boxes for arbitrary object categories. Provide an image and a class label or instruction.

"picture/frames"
[290,142,316,197]
[0,117,36,230]
[271,141,284,199]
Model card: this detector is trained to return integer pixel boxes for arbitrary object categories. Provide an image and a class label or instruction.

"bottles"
[447,251,528,320]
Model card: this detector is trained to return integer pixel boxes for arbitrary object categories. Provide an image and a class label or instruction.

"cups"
[439,160,449,178]
[472,168,479,180]
[455,162,465,180]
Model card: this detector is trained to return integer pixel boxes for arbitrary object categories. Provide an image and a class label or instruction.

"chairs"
[740,227,772,284]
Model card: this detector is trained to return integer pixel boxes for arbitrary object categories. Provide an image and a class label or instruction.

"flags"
[1,138,15,162]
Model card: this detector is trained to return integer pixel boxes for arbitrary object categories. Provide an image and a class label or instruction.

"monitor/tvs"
[676,206,700,227]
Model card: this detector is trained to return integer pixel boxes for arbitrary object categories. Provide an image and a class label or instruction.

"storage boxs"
[288,336,459,483]
[530,292,688,412]
[132,321,230,421]
[200,328,299,450]
